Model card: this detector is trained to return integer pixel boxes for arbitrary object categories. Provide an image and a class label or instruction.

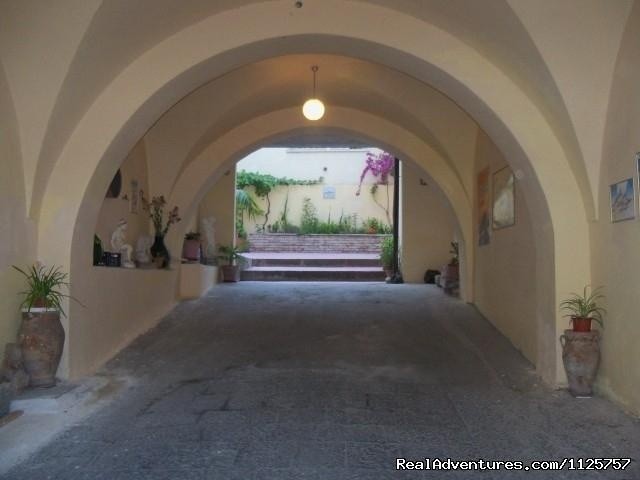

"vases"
[559,331,601,399]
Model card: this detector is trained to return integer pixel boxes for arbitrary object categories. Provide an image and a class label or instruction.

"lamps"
[303,65,325,121]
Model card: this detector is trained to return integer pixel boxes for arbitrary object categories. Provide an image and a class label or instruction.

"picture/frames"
[491,165,515,231]
[632,150,640,208]
[608,176,637,223]
[128,191,140,215]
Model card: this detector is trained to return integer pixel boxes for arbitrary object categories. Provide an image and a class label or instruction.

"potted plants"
[216,241,250,282]
[8,262,86,387]
[183,231,201,261]
[236,189,266,247]
[139,190,182,269]
[368,216,378,234]
[381,235,400,283]
[559,284,608,331]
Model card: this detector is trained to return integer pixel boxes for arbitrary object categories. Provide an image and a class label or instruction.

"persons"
[109,219,135,263]
